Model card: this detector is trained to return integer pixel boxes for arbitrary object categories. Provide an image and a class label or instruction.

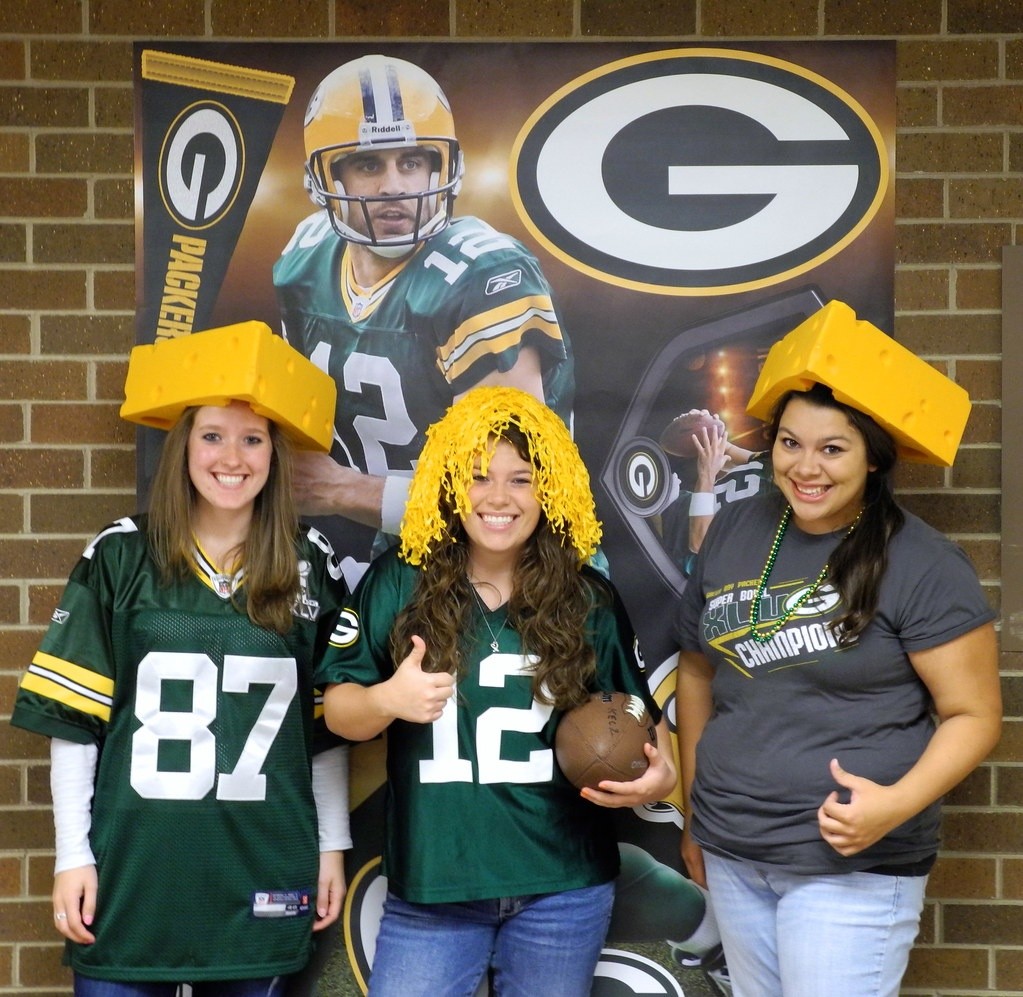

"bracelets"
[686,492,718,519]
[380,476,417,535]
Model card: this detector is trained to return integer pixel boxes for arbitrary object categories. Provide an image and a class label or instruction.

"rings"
[55,913,66,920]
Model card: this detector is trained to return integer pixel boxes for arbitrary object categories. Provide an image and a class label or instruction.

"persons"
[271,54,738,997]
[648,409,774,580]
[312,387,679,997]
[666,382,1003,997]
[10,398,383,997]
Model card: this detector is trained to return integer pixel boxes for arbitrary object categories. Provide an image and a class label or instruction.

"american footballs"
[556,690,656,790]
[660,411,727,458]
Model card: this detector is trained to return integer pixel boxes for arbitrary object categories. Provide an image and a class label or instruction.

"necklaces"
[466,571,511,652]
[749,502,866,643]
